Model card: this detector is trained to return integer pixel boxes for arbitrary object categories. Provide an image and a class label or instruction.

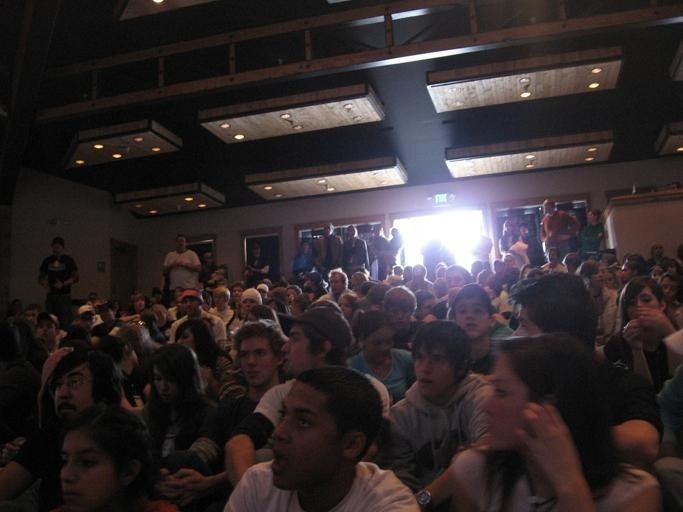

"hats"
[241,288,263,306]
[257,284,269,293]
[277,306,352,345]
[452,283,491,312]
[176,290,205,305]
[37,312,59,329]
[78,304,95,315]
